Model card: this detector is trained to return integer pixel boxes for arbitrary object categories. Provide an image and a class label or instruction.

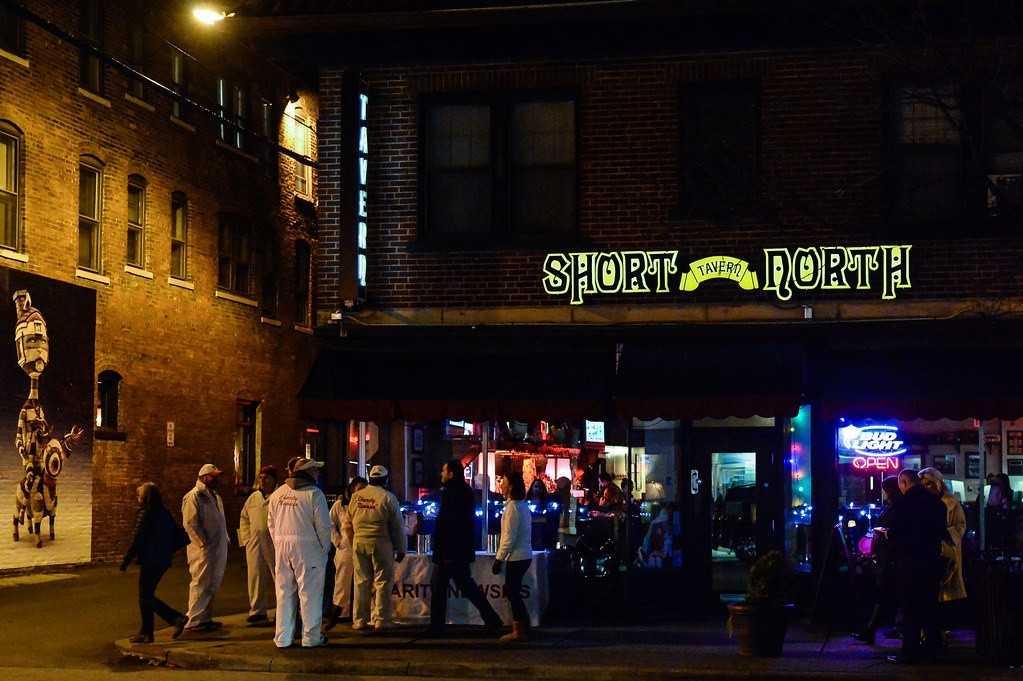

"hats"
[292,458,325,472]
[259,465,278,480]
[370,464,389,478]
[198,464,224,478]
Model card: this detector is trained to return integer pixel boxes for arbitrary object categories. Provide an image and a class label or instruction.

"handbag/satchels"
[170,516,192,551]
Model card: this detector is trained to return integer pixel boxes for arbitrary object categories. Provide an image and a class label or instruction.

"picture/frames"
[965,452,986,480]
[1006,429,1023,455]
[932,455,956,474]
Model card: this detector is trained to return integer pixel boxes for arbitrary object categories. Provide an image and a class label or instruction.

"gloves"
[492,559,503,575]
[119,562,128,572]
[394,553,406,563]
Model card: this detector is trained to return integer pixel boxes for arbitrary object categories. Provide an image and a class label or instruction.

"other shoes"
[324,605,343,632]
[416,630,442,640]
[247,613,267,622]
[886,652,919,665]
[302,636,329,649]
[129,633,154,644]
[883,626,904,641]
[479,619,504,636]
[191,621,223,633]
[172,615,189,639]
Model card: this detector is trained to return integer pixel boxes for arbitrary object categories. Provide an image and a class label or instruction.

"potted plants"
[728,547,790,657]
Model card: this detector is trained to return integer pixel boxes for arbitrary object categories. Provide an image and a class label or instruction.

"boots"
[849,620,880,645]
[499,618,531,644]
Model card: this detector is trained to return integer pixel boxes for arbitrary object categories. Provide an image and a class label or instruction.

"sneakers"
[351,622,368,631]
[374,619,400,630]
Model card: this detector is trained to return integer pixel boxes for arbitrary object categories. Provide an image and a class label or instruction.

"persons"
[424,458,504,636]
[120,483,189,643]
[341,465,405,629]
[268,457,331,647]
[329,477,367,621]
[491,473,534,641]
[239,465,279,623]
[526,473,634,577]
[851,467,968,662]
[182,464,230,631]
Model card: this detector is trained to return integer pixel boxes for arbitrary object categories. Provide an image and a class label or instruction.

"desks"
[375,551,552,630]
